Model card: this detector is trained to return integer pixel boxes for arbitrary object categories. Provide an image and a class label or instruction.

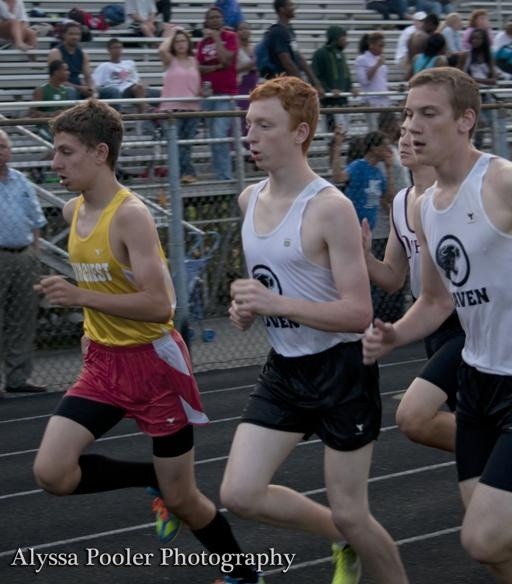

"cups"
[199,81,208,89]
[351,82,361,92]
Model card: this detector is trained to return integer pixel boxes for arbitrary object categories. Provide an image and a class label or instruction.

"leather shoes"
[4,380,49,394]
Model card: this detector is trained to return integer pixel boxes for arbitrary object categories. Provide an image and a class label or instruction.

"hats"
[326,25,347,42]
[412,10,427,21]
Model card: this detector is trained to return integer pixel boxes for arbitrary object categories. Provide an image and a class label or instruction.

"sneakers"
[146,485,184,546]
[328,540,363,584]
[213,572,266,584]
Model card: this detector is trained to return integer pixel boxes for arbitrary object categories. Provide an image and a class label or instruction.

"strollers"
[166,230,221,346]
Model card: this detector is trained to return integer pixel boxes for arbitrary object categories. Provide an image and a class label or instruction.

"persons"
[369,108,413,329]
[356,102,467,449]
[1,128,52,398]
[32,96,263,583]
[325,119,397,330]
[213,73,414,583]
[360,66,511,583]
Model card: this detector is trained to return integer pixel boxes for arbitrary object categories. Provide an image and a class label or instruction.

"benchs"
[1,0,512,205]
